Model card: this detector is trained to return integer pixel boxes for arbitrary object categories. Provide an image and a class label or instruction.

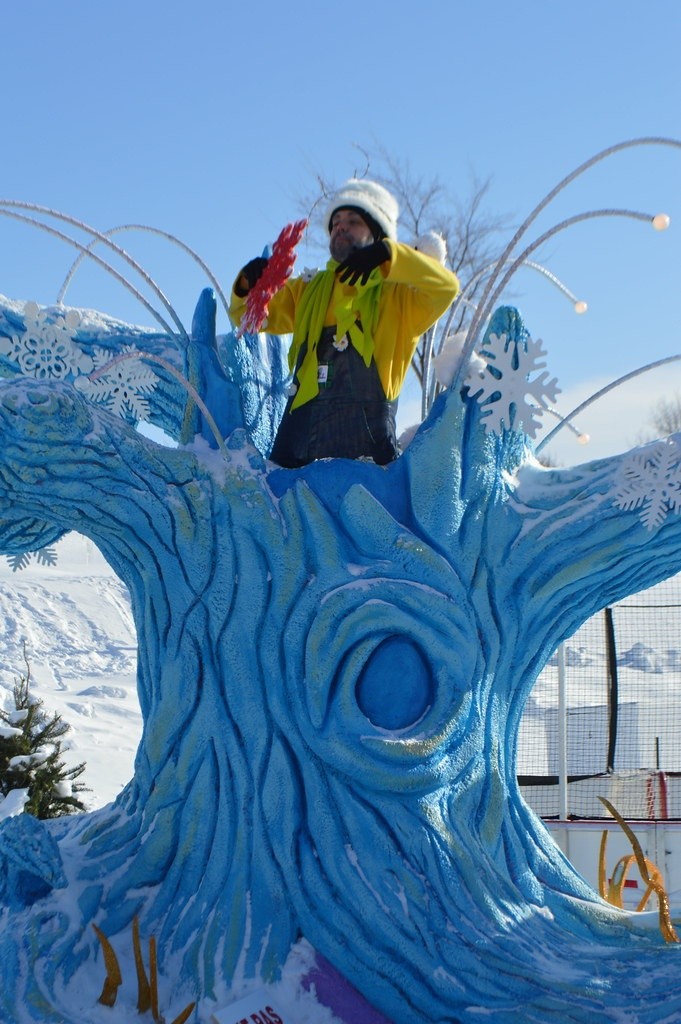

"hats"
[324,180,400,241]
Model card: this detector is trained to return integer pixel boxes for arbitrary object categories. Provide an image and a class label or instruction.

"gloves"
[336,241,390,286]
[243,257,268,288]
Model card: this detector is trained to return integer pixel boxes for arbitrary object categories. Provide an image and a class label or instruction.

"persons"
[228,178,461,470]
